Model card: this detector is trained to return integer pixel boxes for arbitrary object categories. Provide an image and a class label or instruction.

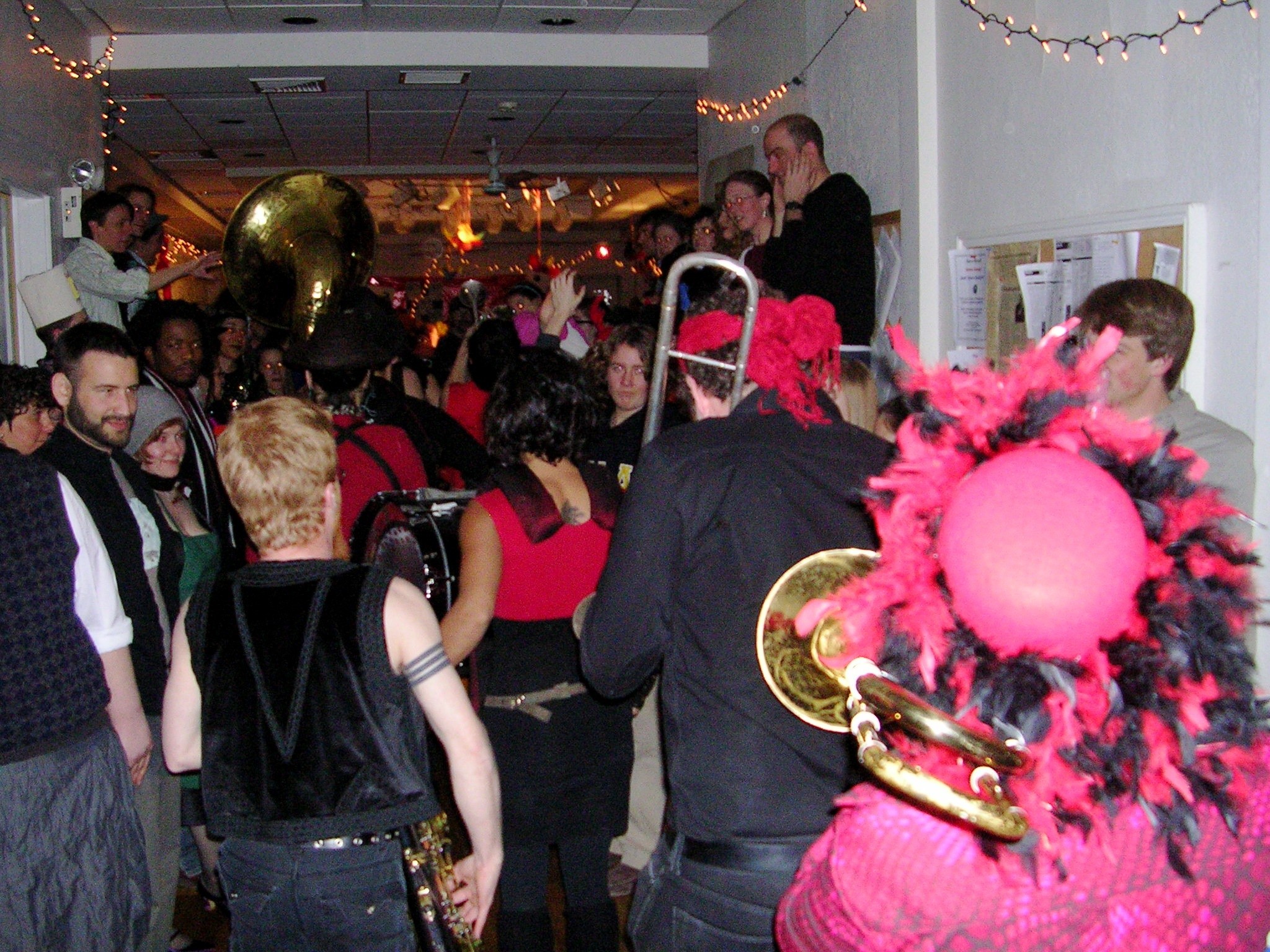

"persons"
[1077,277,1257,660]
[304,321,428,561]
[161,397,503,952]
[65,191,225,331]
[0,114,921,952]
[776,323,1270,952]
[581,281,903,952]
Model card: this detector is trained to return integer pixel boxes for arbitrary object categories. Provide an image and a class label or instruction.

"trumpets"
[402,812,482,952]
[458,280,488,328]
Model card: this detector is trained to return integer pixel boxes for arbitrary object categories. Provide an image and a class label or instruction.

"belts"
[298,827,402,850]
[660,827,813,874]
[483,681,586,722]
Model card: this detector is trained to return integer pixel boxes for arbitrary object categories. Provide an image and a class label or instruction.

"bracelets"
[786,202,802,211]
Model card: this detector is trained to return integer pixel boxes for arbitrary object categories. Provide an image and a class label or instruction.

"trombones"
[572,251,760,641]
[755,547,1035,841]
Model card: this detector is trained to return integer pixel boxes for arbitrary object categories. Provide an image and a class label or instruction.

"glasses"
[724,195,757,213]
[690,227,715,237]
[328,466,346,484]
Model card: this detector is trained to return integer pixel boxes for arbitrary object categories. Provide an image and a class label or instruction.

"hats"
[123,385,187,457]
[144,211,168,230]
[294,310,387,372]
[803,312,1264,881]
[16,263,84,331]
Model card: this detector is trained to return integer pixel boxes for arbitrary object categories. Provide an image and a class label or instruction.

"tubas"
[221,169,379,343]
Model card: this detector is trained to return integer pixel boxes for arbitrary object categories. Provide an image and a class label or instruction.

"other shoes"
[196,877,231,919]
[166,929,193,951]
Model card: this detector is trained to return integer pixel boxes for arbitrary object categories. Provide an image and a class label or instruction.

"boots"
[565,902,621,952]
[495,905,554,952]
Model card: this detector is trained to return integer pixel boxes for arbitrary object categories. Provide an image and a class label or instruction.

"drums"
[348,488,481,672]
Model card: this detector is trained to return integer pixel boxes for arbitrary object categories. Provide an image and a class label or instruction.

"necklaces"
[145,471,179,492]
